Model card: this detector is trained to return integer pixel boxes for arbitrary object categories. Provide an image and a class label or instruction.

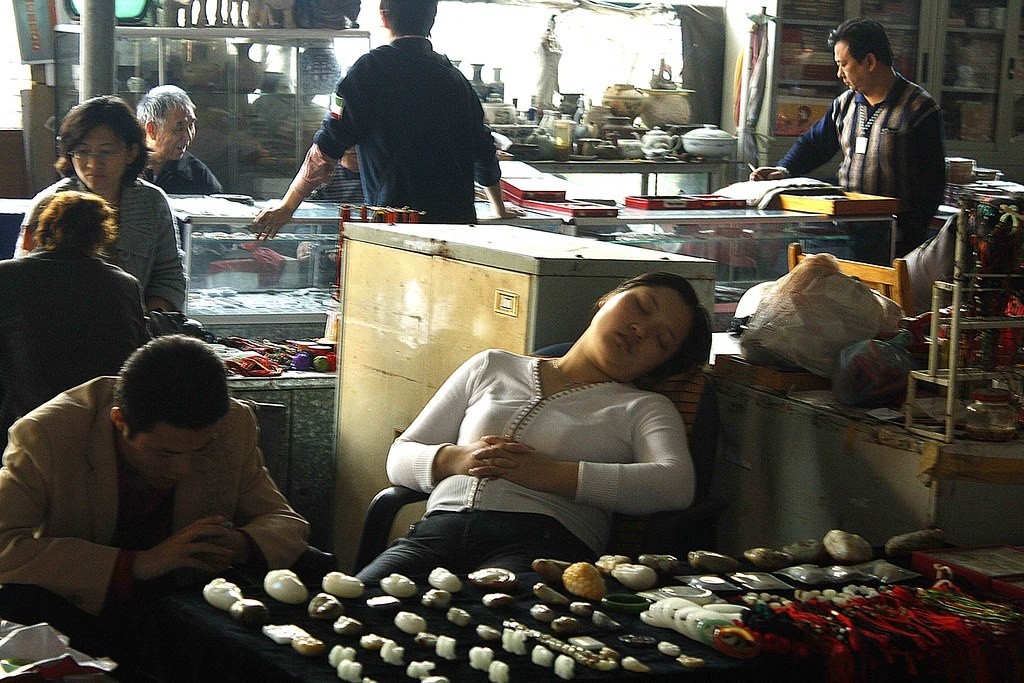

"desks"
[173,542,1023,682]
[706,331,1024,557]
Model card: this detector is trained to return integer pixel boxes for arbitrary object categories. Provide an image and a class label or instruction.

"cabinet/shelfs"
[201,339,334,553]
[720,1,937,181]
[932,0,1024,184]
[165,193,563,341]
[53,24,371,199]
[527,160,719,200]
[335,221,717,576]
[905,200,1023,443]
[507,201,898,332]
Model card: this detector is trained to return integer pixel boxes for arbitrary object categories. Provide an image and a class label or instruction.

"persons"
[250,1,525,244]
[134,85,222,193]
[11,96,186,313]
[748,16,946,264]
[0,336,311,620]
[354,272,711,590]
[0,191,153,463]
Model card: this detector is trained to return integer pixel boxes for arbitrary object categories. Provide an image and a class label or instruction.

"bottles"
[538,110,571,135]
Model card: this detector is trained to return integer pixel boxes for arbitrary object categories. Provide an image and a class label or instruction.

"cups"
[552,120,570,147]
[576,139,602,154]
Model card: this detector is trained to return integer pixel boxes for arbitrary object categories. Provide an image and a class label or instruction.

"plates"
[569,155,597,161]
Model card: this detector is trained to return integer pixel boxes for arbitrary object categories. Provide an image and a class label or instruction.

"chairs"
[788,242,916,316]
[352,343,719,576]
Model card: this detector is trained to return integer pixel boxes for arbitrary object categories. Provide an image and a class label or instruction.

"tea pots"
[630,125,679,160]
[590,141,621,158]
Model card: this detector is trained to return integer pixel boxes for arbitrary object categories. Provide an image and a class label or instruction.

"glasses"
[67,149,129,163]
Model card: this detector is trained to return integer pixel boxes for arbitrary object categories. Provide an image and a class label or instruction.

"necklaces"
[553,359,599,388]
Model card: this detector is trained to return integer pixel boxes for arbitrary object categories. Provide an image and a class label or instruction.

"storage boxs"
[960,103,994,141]
[774,95,835,136]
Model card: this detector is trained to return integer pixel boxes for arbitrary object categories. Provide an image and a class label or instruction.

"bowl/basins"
[617,139,645,158]
[681,124,740,161]
